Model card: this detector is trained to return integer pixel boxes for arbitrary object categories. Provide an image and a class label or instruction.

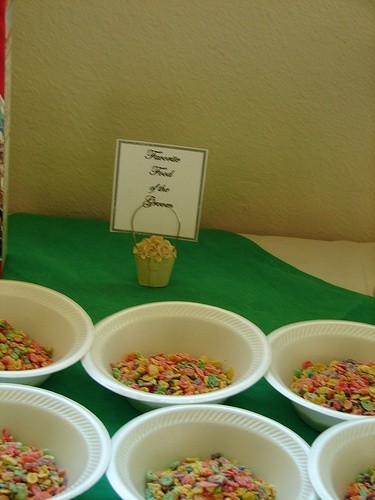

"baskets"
[130,202,181,288]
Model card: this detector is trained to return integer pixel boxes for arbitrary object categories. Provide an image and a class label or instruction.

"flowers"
[134,234,178,260]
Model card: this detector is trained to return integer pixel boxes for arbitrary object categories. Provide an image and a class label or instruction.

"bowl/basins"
[0,280,375,500]
